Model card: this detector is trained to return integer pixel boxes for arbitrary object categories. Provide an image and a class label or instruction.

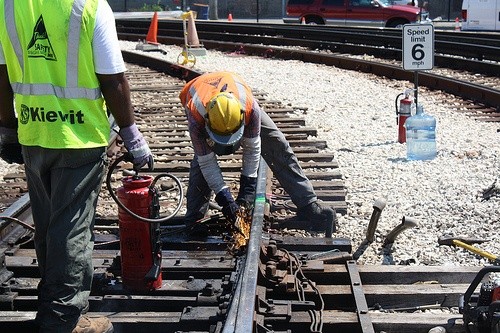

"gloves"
[212,186,241,224]
[120,121,154,172]
[0,117,26,167]
[234,174,258,210]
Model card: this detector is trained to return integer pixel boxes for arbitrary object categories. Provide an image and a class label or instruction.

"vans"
[460,0,500,34]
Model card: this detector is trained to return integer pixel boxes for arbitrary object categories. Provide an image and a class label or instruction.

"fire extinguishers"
[398,88,417,143]
[117,174,163,289]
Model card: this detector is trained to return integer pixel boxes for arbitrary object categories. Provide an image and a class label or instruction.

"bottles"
[404,106,436,160]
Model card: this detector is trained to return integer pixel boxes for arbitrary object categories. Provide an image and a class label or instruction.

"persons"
[178,70,337,234]
[1,0,154,333]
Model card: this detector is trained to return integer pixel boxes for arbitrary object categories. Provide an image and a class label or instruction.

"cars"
[282,0,429,31]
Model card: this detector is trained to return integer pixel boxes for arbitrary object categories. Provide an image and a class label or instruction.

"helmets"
[205,92,248,144]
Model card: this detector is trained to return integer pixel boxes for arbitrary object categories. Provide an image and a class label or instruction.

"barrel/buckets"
[191,4,209,20]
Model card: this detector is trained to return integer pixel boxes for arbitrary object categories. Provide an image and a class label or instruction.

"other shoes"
[185,223,212,237]
[297,199,339,220]
[71,313,114,333]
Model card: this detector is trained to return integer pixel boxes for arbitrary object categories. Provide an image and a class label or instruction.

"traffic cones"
[187,10,205,48]
[227,13,233,21]
[452,16,460,31]
[143,11,159,46]
[300,17,307,25]
